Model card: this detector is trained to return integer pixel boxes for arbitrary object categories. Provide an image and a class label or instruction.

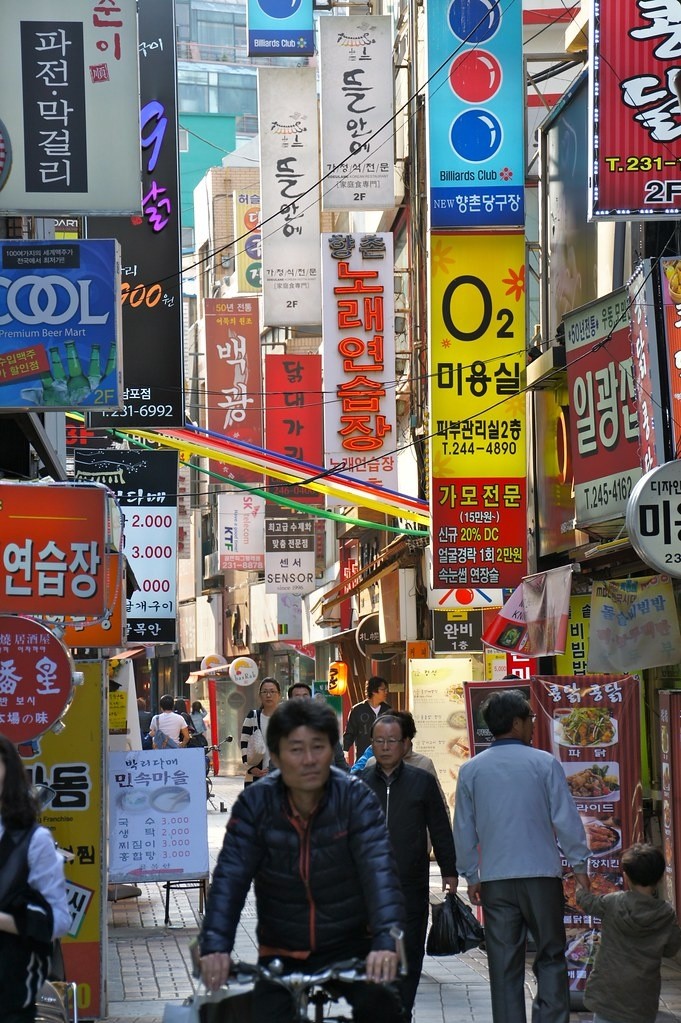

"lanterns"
[328,661,348,697]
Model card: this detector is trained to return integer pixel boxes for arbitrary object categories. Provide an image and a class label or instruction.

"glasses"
[371,736,403,745]
[517,714,537,723]
[260,690,280,695]
[378,688,389,692]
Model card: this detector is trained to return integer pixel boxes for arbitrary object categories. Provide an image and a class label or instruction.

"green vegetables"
[585,765,620,791]
[560,707,615,747]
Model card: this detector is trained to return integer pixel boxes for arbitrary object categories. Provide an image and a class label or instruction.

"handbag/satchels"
[153,728,179,749]
[426,893,485,956]
[247,710,266,766]
[161,973,252,1023]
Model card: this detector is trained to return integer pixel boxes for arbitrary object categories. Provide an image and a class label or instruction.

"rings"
[383,957,390,962]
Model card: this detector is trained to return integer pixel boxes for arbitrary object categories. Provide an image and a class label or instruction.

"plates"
[582,824,620,855]
[554,716,617,748]
[566,788,617,799]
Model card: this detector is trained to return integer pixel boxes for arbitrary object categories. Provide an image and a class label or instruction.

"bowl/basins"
[117,789,150,813]
[150,787,190,812]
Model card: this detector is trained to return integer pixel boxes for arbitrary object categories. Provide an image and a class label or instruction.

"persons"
[454,690,593,1023]
[240,676,281,789]
[356,715,459,1012]
[149,694,190,749]
[173,699,197,748]
[288,683,349,768]
[137,697,155,751]
[575,843,681,1023]
[0,734,72,1023]
[342,675,392,761]
[188,701,208,735]
[350,709,452,854]
[200,697,408,1023]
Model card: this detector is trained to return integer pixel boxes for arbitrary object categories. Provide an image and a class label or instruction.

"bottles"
[38,339,116,407]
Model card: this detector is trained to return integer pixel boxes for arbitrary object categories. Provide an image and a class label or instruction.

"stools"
[163,880,207,924]
